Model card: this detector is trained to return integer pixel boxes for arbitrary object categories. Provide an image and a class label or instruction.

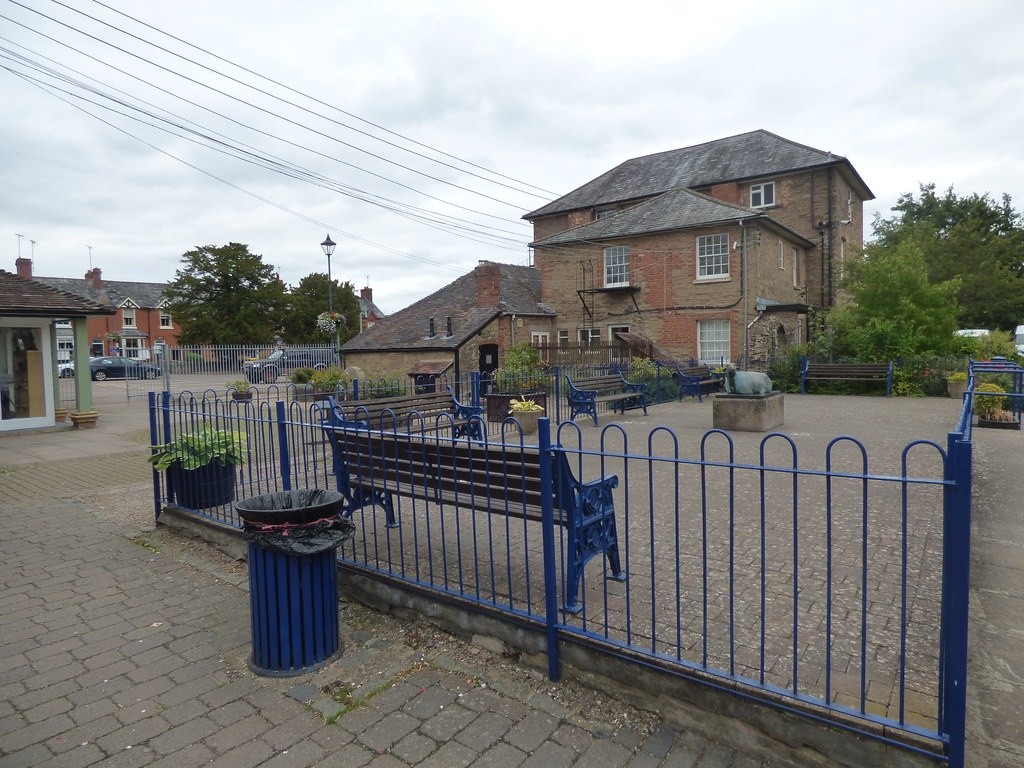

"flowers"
[317,312,346,335]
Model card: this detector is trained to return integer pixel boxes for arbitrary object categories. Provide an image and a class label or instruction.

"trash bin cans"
[233,487,358,679]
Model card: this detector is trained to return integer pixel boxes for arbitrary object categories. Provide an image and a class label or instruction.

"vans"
[1014,324,1024,359]
[952,329,991,341]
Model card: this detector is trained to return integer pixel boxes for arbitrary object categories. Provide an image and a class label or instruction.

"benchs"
[322,419,628,616]
[674,362,725,403]
[800,359,894,397]
[326,385,483,448]
[564,368,649,428]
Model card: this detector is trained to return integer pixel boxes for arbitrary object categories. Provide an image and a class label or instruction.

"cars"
[84,356,162,381]
[56,356,95,378]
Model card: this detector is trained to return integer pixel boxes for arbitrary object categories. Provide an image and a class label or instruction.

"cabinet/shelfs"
[12,350,44,418]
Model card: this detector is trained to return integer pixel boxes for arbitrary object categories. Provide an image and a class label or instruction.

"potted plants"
[508,394,545,436]
[291,366,314,403]
[311,367,347,401]
[483,343,550,424]
[226,380,253,403]
[147,424,248,510]
[945,371,968,399]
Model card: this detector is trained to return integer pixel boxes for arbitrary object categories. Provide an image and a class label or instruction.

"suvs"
[238,347,336,383]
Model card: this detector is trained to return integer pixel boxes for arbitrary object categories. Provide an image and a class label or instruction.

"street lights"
[319,232,337,347]
